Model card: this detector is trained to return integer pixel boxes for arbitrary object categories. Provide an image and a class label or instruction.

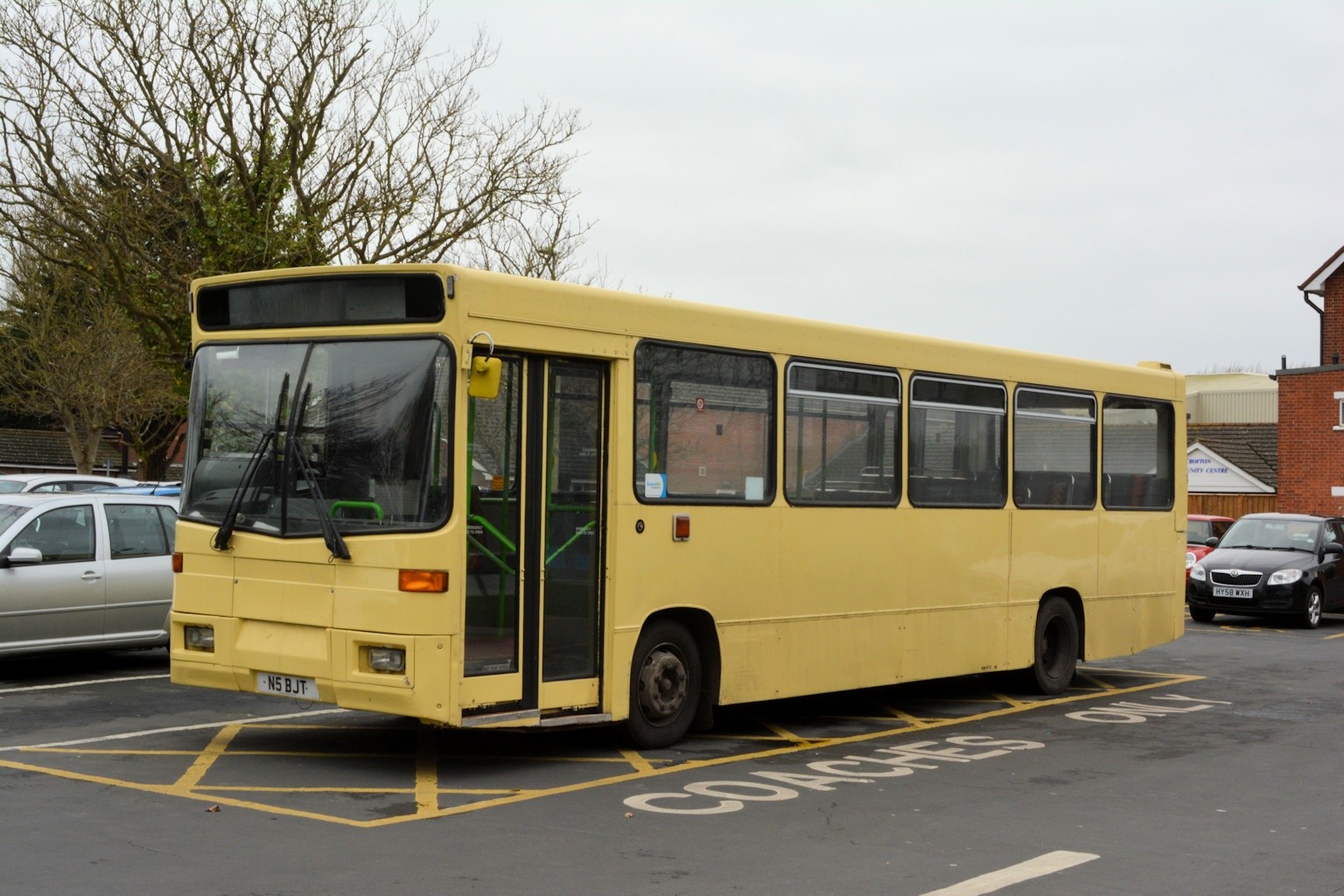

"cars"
[1189,513,1344,624]
[0,446,305,668]
[1184,513,1240,596]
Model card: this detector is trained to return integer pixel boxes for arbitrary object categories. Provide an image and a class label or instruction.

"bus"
[167,261,1190,750]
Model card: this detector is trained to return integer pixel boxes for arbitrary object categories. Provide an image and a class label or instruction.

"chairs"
[57,525,91,560]
[1205,525,1220,540]
[1188,531,1198,540]
[811,465,1158,509]
[1302,529,1317,552]
